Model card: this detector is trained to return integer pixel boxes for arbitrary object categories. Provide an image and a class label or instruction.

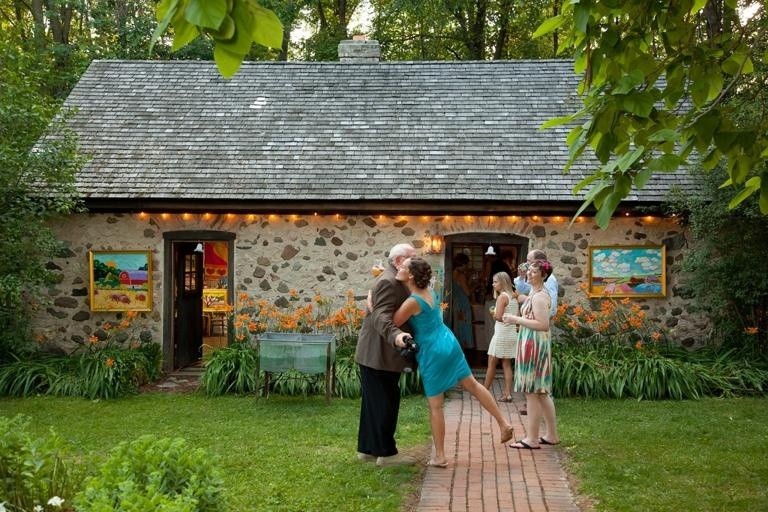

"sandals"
[500,425,514,445]
[427,457,450,467]
[497,394,513,402]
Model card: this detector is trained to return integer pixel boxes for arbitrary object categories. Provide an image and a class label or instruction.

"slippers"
[510,437,561,449]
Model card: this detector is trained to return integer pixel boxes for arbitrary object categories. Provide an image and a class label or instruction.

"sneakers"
[520,403,527,415]
[358,452,417,466]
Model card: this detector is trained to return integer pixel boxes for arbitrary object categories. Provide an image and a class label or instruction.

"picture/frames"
[89,249,152,312]
[202,289,228,312]
[588,245,667,298]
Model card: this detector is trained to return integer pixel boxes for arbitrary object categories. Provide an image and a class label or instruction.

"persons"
[484,250,516,367]
[468,271,522,403]
[453,251,476,364]
[364,255,515,469]
[347,244,418,470]
[510,248,559,415]
[501,258,564,449]
[513,258,531,296]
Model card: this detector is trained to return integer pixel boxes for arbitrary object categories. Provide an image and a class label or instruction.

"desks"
[257,333,336,405]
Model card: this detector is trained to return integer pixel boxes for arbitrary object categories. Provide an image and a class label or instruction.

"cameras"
[401,337,419,372]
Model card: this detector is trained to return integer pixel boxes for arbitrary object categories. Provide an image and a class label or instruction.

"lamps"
[193,243,203,252]
[486,233,495,255]
